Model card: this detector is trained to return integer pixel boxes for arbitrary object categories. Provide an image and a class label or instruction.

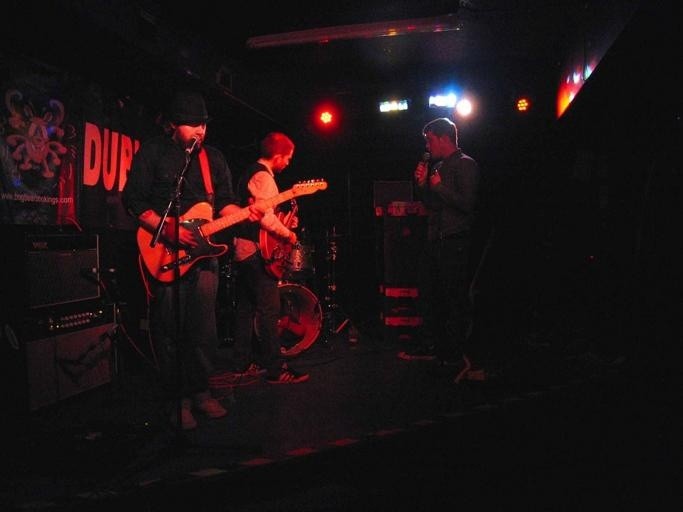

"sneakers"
[170,402,197,430]
[399,343,439,361]
[264,368,309,384]
[197,396,228,418]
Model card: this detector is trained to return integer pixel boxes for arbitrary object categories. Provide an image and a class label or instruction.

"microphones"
[184,134,200,154]
[83,270,106,290]
[78,332,107,361]
[416,152,431,182]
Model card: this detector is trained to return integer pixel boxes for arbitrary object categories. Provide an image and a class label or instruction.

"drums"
[287,244,313,273]
[253,281,323,357]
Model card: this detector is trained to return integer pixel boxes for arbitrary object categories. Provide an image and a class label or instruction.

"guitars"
[260,198,299,281]
[136,179,328,283]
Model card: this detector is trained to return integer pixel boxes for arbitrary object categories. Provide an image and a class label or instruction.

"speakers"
[20,232,101,311]
[17,323,122,413]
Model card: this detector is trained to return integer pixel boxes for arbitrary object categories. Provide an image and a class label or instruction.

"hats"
[167,87,209,123]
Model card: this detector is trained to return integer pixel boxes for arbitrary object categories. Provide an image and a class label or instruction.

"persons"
[118,89,269,435]
[396,115,490,384]
[227,132,312,385]
